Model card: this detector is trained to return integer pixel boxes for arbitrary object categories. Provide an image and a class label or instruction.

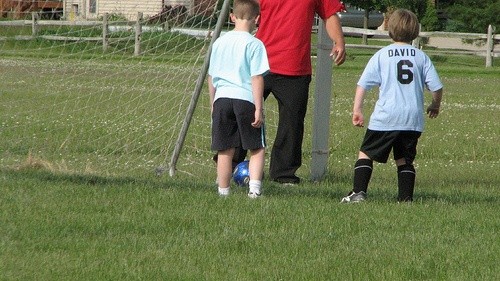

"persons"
[212,0,345,185]
[208,0,271,199]
[341,8,443,204]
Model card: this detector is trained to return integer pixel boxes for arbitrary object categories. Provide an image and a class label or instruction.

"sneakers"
[341,189,366,202]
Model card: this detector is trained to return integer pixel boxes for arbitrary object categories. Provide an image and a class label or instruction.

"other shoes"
[248,192,261,199]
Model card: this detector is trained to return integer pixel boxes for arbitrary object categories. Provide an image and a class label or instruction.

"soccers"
[232,160,264,187]
